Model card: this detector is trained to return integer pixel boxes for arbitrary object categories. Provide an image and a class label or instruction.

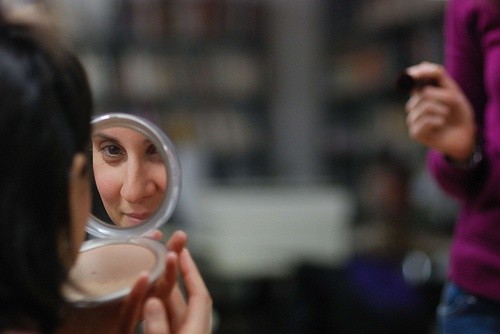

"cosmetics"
[57,111,182,307]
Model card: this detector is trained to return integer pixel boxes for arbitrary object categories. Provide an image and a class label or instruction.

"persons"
[90,127,167,228]
[1,31,214,334]
[410,1,500,334]
